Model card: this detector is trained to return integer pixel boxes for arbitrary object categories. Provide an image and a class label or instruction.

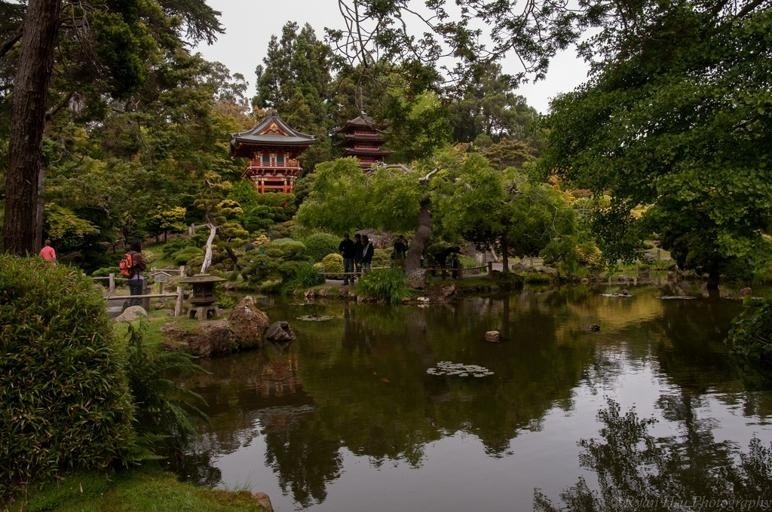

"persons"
[452,255,459,279]
[361,236,373,269]
[339,233,355,285]
[128,240,147,306]
[353,234,364,277]
[39,239,57,263]
[342,306,359,356]
[394,235,409,259]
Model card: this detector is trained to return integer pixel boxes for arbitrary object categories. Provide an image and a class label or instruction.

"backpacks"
[119,250,138,279]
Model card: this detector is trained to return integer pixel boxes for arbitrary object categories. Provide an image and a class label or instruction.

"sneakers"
[341,282,354,286]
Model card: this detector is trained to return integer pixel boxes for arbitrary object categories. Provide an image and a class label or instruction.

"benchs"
[318,271,364,285]
